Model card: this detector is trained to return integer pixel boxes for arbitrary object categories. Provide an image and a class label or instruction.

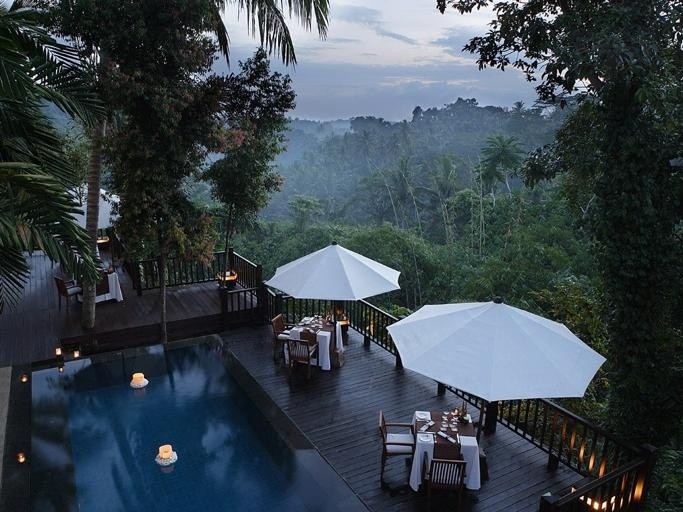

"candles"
[158,445,172,459]
[133,372,144,384]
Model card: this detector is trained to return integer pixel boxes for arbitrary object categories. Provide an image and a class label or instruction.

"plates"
[420,435,432,441]
[416,412,429,419]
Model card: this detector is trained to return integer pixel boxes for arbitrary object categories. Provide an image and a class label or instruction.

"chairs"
[54,276,83,314]
[378,408,469,512]
[271,312,320,381]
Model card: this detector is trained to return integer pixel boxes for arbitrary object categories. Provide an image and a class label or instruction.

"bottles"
[454,407,459,415]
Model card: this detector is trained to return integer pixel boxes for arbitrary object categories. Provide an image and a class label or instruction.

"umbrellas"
[261,241,402,349]
[385,296,605,441]
[53,182,124,232]
